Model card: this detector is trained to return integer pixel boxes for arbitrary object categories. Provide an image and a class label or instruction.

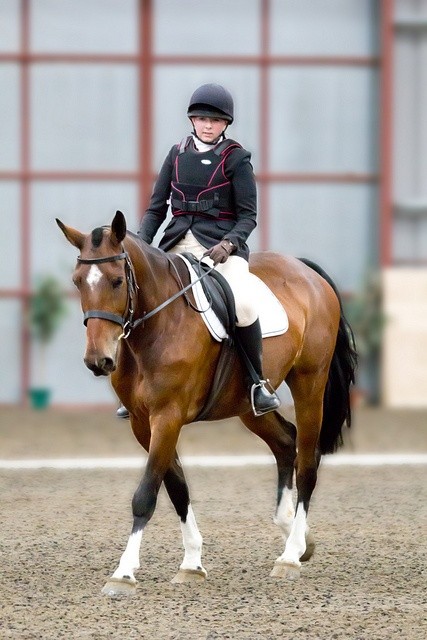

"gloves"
[203,240,236,267]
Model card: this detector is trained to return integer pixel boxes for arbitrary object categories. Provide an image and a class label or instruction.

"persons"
[115,83,281,419]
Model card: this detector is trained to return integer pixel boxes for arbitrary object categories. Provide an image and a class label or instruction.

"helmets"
[187,82,234,125]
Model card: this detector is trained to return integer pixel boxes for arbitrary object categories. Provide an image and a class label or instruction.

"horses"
[54,210,358,598]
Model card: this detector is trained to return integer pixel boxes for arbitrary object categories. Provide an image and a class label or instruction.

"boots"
[235,318,280,412]
